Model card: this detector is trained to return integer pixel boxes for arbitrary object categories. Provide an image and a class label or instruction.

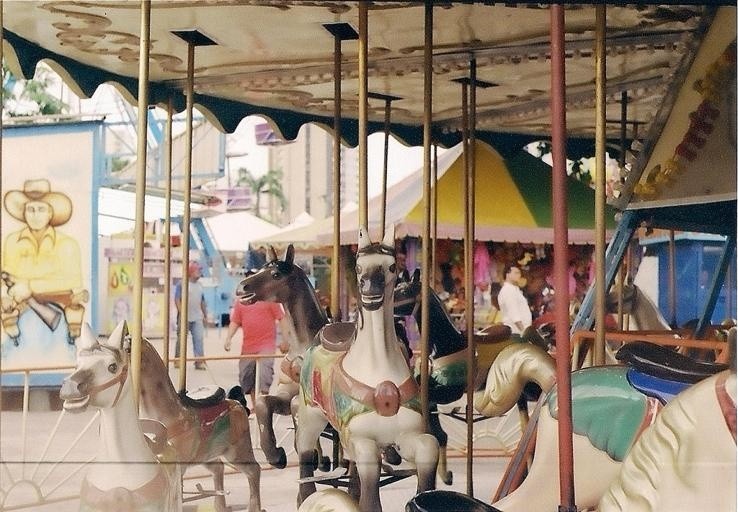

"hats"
[189,264,201,275]
[4,180,72,226]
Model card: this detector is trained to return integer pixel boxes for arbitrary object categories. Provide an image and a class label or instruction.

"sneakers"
[196,362,206,369]
[174,362,179,368]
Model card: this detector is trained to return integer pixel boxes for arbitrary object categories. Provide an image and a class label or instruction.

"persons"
[0,179,88,339]
[175,260,209,370]
[497,264,533,337]
[224,268,291,413]
